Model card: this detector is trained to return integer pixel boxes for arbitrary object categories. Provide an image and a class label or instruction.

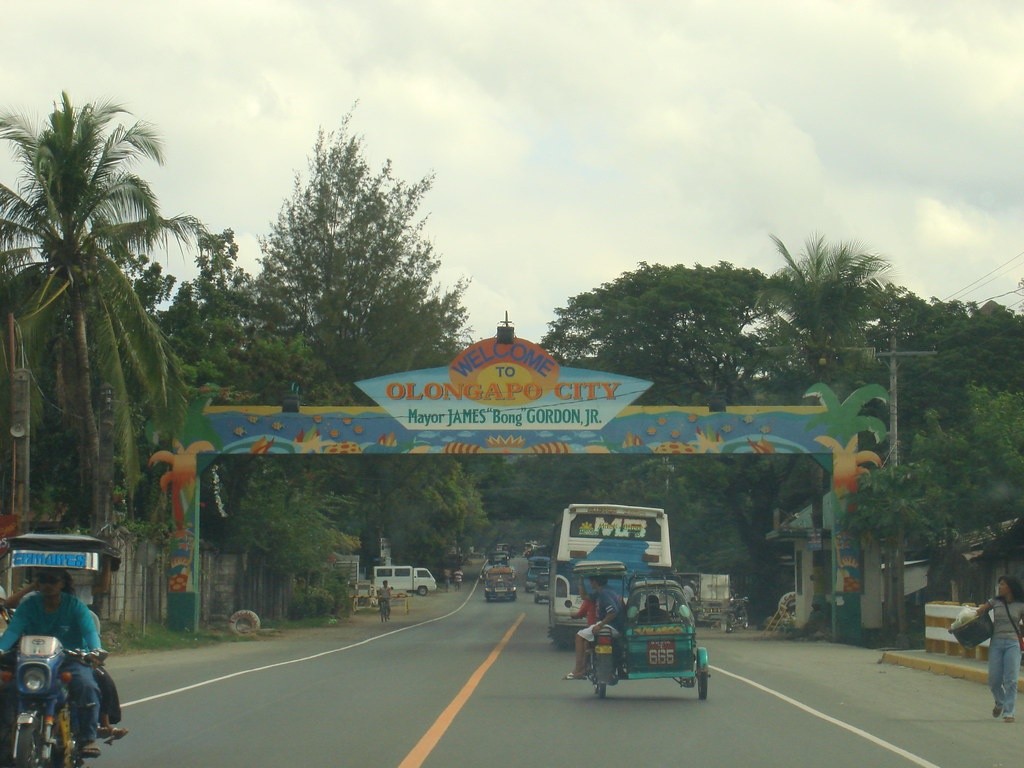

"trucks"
[373,565,437,596]
[672,572,729,628]
[525,556,550,593]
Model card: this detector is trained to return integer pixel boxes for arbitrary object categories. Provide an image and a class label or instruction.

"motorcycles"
[574,559,711,701]
[718,597,751,633]
[0,533,122,768]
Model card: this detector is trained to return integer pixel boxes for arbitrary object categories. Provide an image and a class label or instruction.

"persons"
[974,576,1024,723]
[636,594,672,623]
[376,580,392,620]
[0,567,129,759]
[561,576,627,681]
[728,593,749,621]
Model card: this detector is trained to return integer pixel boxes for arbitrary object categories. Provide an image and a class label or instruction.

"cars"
[534,573,550,603]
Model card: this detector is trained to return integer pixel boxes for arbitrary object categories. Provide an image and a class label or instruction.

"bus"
[551,504,672,653]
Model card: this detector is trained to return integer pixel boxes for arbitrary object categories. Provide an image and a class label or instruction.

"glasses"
[39,576,62,585]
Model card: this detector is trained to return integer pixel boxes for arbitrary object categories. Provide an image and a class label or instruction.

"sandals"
[79,746,100,758]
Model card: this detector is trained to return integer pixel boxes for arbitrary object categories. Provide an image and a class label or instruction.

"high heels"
[104,728,128,745]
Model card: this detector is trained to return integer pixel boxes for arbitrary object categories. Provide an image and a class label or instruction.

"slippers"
[564,672,587,680]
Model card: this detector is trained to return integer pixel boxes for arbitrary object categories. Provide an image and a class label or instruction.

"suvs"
[491,542,547,567]
[484,567,516,602]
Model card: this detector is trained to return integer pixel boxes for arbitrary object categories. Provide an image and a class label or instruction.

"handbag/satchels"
[1018,634,1024,651]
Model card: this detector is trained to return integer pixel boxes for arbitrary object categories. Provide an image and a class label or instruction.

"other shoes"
[993,706,1002,717]
[1005,717,1015,722]
